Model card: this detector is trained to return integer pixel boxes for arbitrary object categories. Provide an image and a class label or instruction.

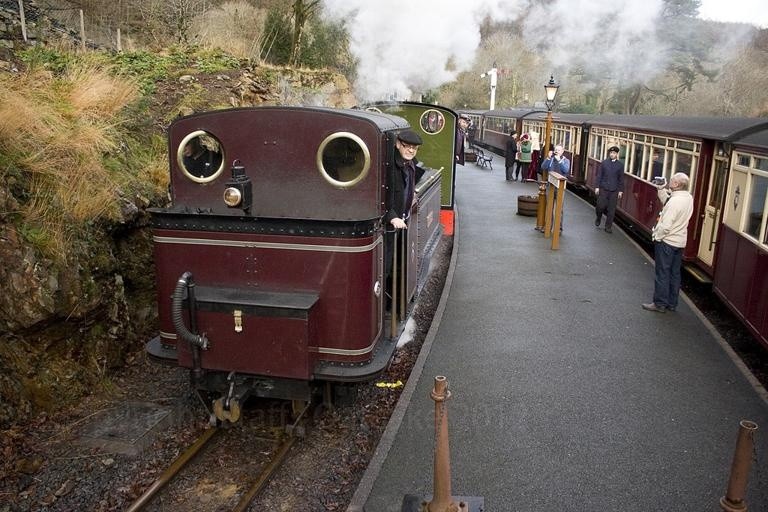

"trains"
[456,107,767,351]
[142,98,457,430]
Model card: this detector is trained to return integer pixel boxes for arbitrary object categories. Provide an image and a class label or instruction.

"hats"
[523,134,528,139]
[459,113,472,123]
[511,131,518,136]
[608,146,619,153]
[398,131,424,145]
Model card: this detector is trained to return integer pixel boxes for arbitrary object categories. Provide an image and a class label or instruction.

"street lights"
[536,76,560,231]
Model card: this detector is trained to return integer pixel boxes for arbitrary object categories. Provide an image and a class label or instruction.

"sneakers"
[604,226,612,233]
[506,177,525,182]
[595,219,601,226]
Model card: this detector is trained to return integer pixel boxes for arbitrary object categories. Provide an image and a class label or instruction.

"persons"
[467,121,477,149]
[592,146,624,233]
[540,144,571,236]
[456,115,471,166]
[541,138,554,162]
[505,131,532,183]
[383,131,423,314]
[183,136,220,179]
[642,173,694,312]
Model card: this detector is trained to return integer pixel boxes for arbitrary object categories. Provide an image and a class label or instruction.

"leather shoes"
[642,303,676,314]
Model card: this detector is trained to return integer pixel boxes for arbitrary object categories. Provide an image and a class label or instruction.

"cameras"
[554,151,559,154]
[653,178,665,185]
